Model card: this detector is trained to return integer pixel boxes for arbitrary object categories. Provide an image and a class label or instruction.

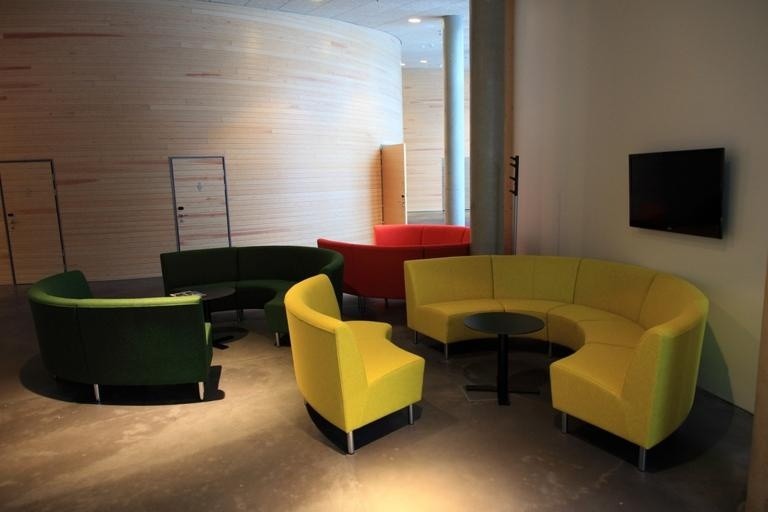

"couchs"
[160,246,344,348]
[402,252,709,473]
[316,223,470,313]
[24,269,213,404]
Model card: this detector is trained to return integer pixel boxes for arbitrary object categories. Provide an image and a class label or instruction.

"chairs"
[282,272,429,456]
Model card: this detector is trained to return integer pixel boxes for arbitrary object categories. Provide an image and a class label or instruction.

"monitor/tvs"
[629,146,725,240]
